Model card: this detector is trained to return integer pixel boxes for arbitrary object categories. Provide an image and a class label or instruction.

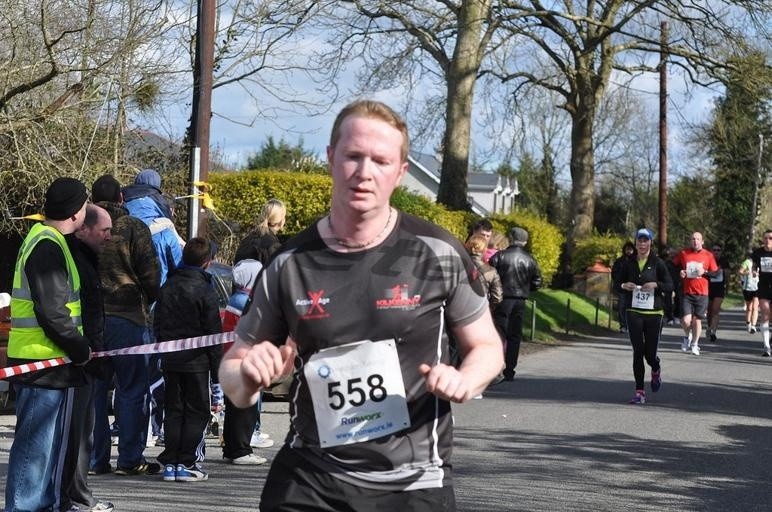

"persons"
[88,175,160,476]
[233,200,286,450]
[71,204,115,512]
[153,238,223,482]
[121,170,184,446]
[445,219,542,387]
[611,230,772,404]
[223,259,267,465]
[4,178,93,512]
[219,100,505,512]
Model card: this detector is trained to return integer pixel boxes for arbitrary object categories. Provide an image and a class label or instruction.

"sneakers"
[690,343,700,356]
[762,347,772,356]
[250,432,274,448]
[87,461,208,482]
[682,333,693,352]
[62,500,114,511]
[746,323,756,332]
[651,368,661,392]
[629,390,647,403]
[223,454,267,464]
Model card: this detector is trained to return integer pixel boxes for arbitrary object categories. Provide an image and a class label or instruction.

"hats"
[636,229,652,240]
[136,169,161,188]
[232,259,263,290]
[45,178,87,220]
[511,227,529,241]
